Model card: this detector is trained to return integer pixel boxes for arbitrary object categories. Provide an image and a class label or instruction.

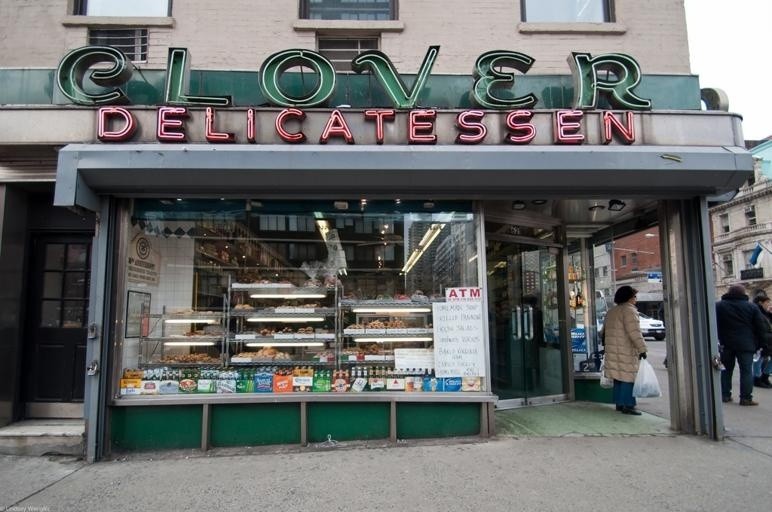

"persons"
[602,285,649,416]
[752,295,772,389]
[715,285,770,406]
[752,287,767,299]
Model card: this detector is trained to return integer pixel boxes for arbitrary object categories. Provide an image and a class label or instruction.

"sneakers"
[740,399,759,405]
[722,396,733,402]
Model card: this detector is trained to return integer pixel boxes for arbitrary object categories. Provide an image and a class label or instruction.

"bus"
[545,288,608,345]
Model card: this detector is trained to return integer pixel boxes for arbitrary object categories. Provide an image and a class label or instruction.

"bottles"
[122,364,437,391]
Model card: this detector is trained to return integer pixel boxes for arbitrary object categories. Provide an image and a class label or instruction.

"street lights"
[645,232,658,241]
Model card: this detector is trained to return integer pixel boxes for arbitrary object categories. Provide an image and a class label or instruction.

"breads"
[152,352,211,363]
[362,342,382,358]
[235,303,252,309]
[345,319,407,329]
[185,325,222,337]
[236,345,292,361]
[298,327,313,333]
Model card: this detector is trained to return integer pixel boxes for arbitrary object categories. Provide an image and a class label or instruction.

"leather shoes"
[616,405,624,411]
[623,408,641,415]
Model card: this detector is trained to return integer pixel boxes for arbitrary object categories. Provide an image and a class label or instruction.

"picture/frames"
[124,290,152,338]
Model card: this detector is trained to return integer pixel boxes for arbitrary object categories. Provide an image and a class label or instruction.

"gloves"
[638,352,647,360]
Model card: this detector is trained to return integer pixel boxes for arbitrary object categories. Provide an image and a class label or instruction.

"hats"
[613,286,639,305]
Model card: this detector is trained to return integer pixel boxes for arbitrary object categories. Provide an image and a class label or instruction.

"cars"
[638,311,666,341]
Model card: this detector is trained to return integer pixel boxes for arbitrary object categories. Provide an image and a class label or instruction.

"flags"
[749,244,767,270]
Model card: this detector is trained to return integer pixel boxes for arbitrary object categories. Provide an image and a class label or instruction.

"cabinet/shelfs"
[138,224,446,373]
